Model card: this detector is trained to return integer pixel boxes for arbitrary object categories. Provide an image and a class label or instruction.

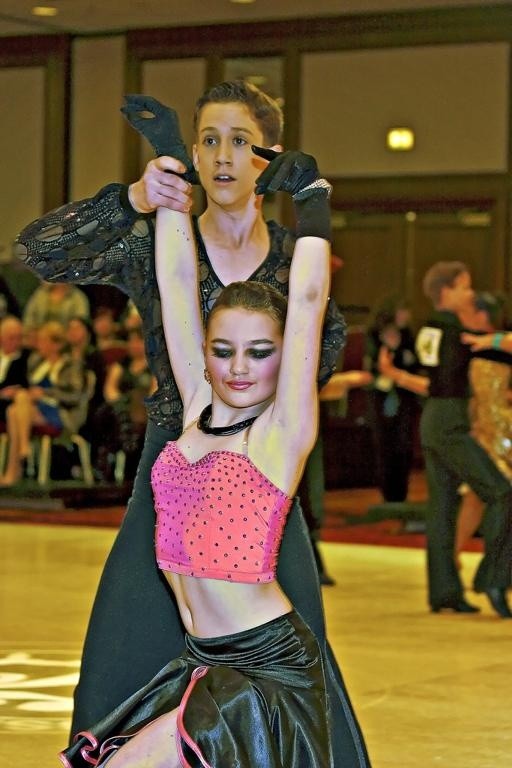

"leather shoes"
[474,577,511,618]
[433,592,481,613]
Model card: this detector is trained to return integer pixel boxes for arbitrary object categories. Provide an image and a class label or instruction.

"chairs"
[0,367,95,485]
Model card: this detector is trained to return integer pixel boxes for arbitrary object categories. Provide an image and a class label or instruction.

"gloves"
[250,144,331,243]
[119,94,197,184]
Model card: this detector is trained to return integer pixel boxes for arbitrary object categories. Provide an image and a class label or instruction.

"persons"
[299,255,346,585]
[0,268,159,489]
[365,294,430,503]
[14,81,372,768]
[417,261,511,619]
[57,93,331,768]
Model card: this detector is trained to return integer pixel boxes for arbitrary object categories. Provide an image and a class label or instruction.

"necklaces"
[198,404,261,437]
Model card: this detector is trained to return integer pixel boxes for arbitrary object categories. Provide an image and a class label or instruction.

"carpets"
[1,499,488,554]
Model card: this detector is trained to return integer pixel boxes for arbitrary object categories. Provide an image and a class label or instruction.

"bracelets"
[293,177,332,200]
